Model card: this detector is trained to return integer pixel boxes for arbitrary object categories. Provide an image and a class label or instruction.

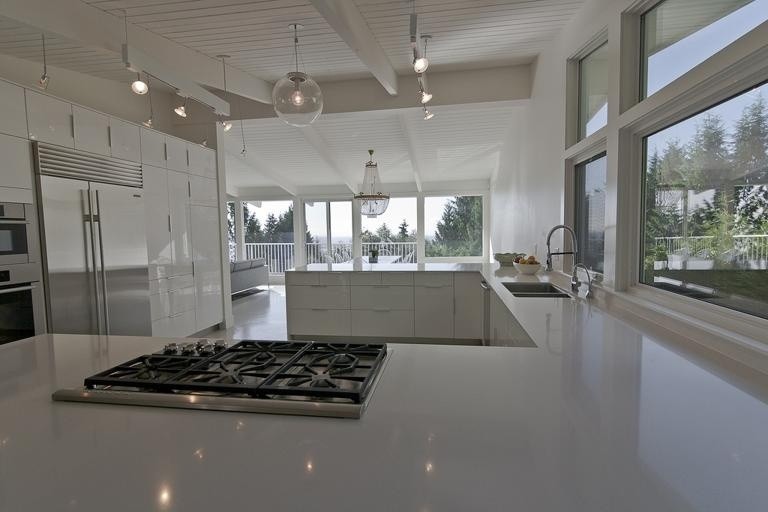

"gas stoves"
[50,340,386,421]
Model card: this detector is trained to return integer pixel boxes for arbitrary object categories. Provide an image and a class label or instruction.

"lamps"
[354,150,390,218]
[142,75,153,128]
[40,34,49,87]
[409,0,434,120]
[121,10,232,132]
[240,120,247,157]
[272,23,323,127]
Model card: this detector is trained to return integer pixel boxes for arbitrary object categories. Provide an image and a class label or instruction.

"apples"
[513,255,538,264]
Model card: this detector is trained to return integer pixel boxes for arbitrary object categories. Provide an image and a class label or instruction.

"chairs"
[324,249,415,263]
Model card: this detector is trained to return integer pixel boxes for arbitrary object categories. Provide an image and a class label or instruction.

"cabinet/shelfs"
[0,77,224,337]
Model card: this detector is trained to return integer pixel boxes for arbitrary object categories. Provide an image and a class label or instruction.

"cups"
[417,261,424,271]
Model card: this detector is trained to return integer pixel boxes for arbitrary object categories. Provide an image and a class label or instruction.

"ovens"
[0,220,31,264]
[1,279,47,345]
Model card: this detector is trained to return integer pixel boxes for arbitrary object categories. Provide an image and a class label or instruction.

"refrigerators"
[31,139,155,337]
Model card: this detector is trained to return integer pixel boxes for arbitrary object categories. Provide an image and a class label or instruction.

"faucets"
[546,224,582,286]
[573,262,594,298]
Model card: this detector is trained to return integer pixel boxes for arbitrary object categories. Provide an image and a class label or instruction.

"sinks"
[513,293,573,298]
[501,281,564,293]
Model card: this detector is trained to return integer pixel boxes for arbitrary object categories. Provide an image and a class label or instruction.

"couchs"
[230,258,270,293]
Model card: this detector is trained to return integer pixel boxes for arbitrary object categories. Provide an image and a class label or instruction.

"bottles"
[327,260,332,271]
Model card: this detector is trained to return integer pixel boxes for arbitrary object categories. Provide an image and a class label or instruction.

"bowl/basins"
[294,263,306,271]
[514,274,540,283]
[512,261,541,274]
[492,252,526,264]
[495,266,515,278]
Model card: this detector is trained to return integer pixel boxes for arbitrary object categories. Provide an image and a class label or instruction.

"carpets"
[232,288,263,301]
